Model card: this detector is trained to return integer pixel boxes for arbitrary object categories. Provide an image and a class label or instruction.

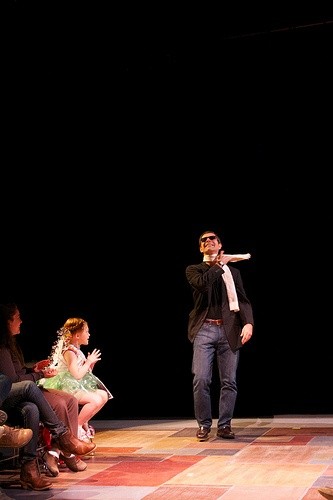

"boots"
[19,458,53,491]
[59,427,97,456]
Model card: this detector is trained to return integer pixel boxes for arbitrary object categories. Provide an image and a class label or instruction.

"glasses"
[200,236,216,242]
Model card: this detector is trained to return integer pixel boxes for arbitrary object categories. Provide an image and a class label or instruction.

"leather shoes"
[216,425,236,439]
[196,426,211,441]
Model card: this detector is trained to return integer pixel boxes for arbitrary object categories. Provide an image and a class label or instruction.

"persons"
[185,231,255,441]
[0,304,113,491]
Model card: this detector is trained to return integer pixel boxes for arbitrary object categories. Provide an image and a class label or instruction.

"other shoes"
[0,410,7,426]
[0,425,33,448]
[42,450,59,477]
[76,420,95,444]
[59,452,88,471]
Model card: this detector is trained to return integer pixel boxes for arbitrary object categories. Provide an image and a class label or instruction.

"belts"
[204,319,222,327]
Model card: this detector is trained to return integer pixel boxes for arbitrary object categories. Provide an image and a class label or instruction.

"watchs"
[218,261,224,267]
